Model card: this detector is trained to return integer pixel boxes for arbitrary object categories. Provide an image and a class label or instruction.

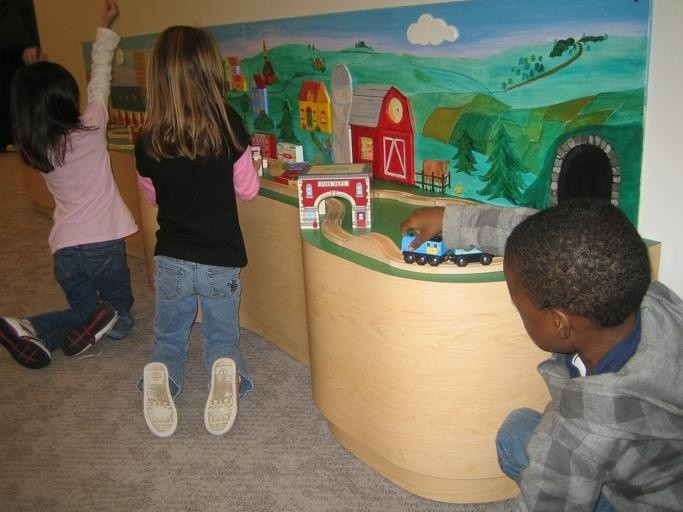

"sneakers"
[203,357,237,436]
[0,315,50,370]
[142,361,177,438]
[63,297,118,357]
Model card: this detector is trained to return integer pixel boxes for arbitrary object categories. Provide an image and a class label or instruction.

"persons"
[135,26,261,437]
[401,198,683,512]
[0,0,140,368]
